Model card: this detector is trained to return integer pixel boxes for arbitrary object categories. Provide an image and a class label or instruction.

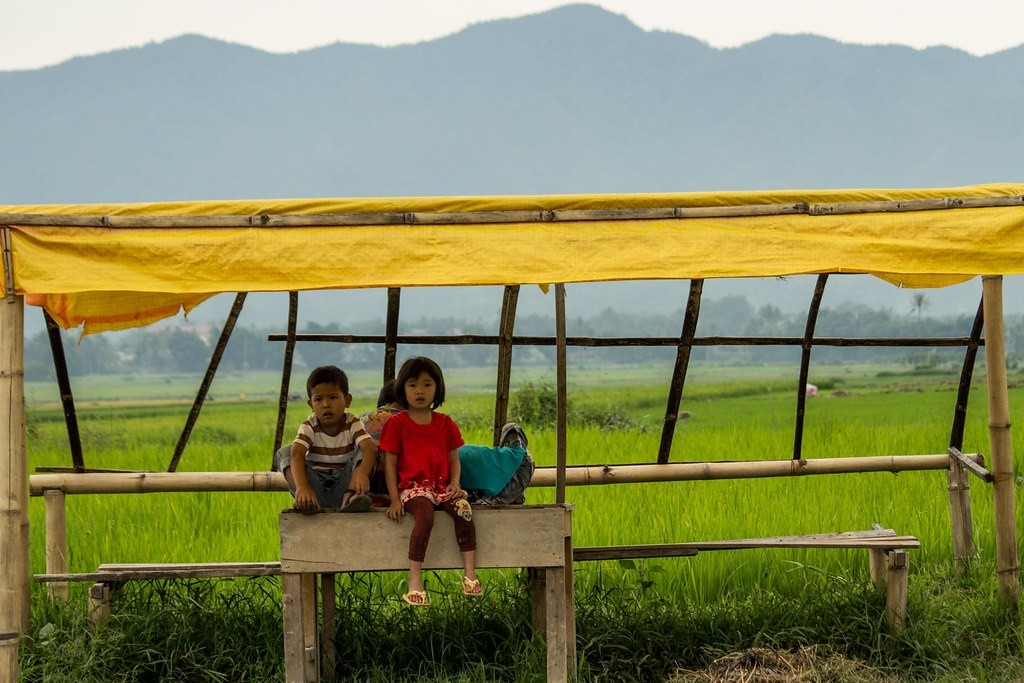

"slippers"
[402,590,430,606]
[461,575,482,596]
[293,501,325,514]
[338,493,372,512]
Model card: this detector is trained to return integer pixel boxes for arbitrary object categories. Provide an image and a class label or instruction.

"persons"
[357,378,407,496]
[448,422,535,507]
[378,355,484,606]
[273,365,377,514]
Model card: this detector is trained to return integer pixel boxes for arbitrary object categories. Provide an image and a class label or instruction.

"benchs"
[273,501,577,683]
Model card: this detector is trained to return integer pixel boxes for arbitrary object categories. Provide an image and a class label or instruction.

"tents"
[0,182,1024,683]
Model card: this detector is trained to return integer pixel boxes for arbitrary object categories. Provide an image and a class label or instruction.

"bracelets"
[450,478,461,486]
